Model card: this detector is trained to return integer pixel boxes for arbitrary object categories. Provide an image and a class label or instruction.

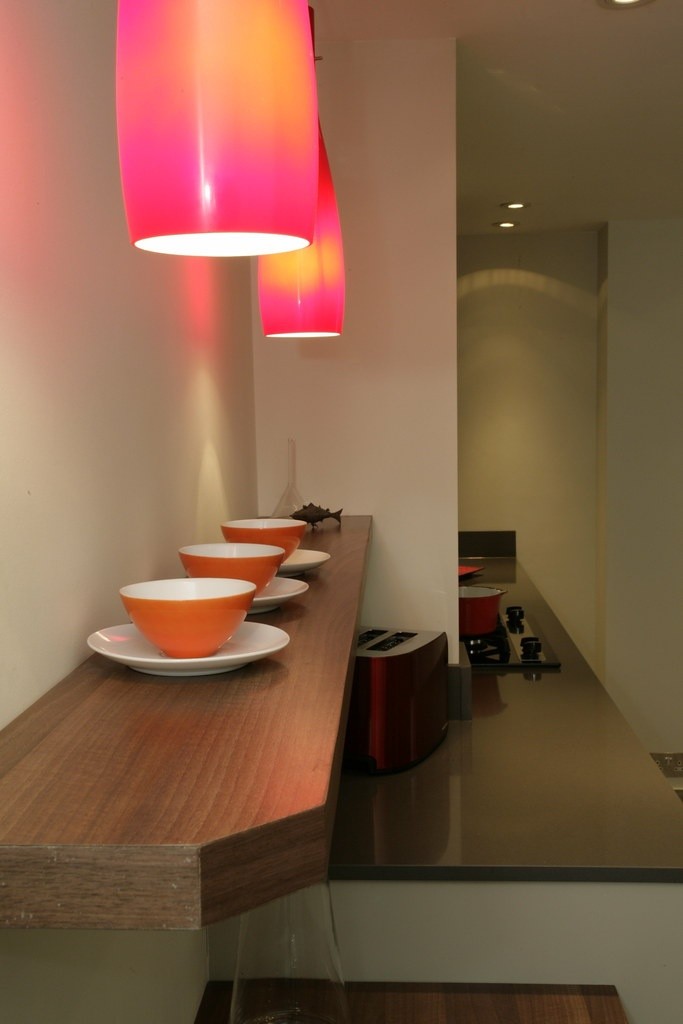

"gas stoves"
[460,605,562,670]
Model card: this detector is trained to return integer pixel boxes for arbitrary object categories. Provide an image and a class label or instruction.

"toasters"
[341,625,450,778]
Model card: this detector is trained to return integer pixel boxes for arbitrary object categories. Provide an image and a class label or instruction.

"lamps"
[115,0,319,258]
[256,116,345,338]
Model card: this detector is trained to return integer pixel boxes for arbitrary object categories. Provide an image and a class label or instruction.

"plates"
[247,577,310,615]
[278,549,331,578]
[86,624,290,677]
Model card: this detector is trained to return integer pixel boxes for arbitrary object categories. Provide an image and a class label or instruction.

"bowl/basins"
[220,518,308,566]
[178,543,285,598]
[117,577,258,659]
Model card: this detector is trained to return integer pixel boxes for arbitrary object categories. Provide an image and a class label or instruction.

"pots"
[459,587,509,636]
